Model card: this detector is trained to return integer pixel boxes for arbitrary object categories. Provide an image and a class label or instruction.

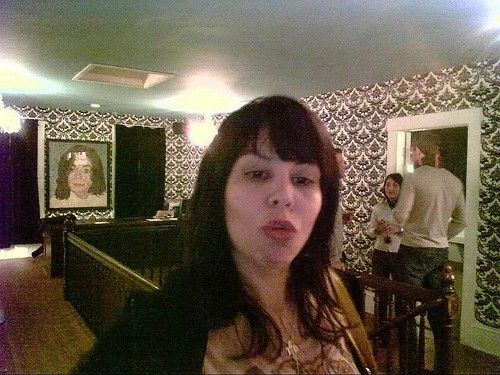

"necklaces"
[283,305,297,361]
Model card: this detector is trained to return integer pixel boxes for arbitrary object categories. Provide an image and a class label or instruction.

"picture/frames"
[45,138,111,212]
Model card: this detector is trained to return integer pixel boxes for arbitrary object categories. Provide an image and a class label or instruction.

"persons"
[80,96,377,375]
[392,131,467,375]
[49,144,107,207]
[331,148,353,270]
[369,173,404,344]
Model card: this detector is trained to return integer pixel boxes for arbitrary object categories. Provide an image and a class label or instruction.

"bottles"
[381,219,392,245]
